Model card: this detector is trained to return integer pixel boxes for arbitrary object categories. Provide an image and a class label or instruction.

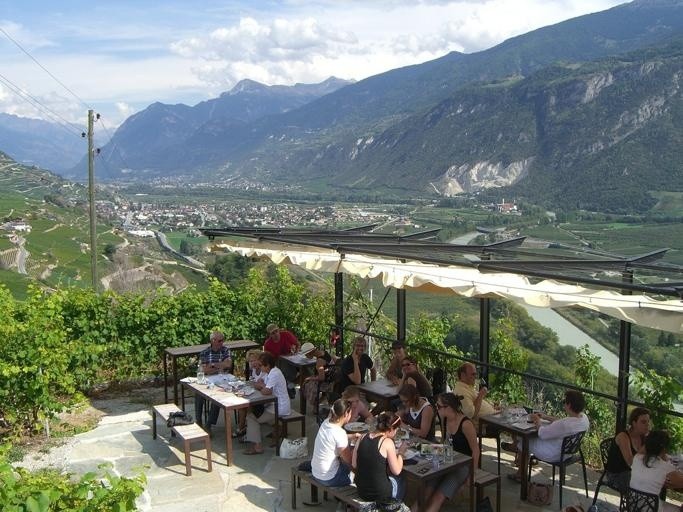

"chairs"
[592,437,628,508]
[530,430,589,510]
[620,485,659,512]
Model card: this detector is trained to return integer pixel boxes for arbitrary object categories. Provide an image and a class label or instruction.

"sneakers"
[288,388,296,398]
[232,425,264,454]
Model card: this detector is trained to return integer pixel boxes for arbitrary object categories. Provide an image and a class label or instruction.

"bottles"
[318,342,324,350]
[217,359,224,376]
[197,360,203,383]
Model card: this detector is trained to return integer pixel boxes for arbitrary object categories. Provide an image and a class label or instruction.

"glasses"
[436,403,448,409]
[390,415,402,428]
[401,399,409,403]
[401,363,414,367]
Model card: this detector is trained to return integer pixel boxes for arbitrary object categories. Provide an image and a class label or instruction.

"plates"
[420,443,442,456]
[235,388,255,396]
[399,428,409,439]
[343,422,367,432]
[226,381,245,388]
[179,376,197,383]
[395,449,415,460]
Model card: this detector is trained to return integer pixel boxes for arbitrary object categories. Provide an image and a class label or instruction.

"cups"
[498,394,543,429]
[671,456,679,467]
[432,437,452,467]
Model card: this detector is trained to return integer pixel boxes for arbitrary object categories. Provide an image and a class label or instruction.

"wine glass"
[478,378,490,394]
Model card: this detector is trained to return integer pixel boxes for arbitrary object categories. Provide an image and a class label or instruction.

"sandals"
[508,473,521,483]
[501,442,518,452]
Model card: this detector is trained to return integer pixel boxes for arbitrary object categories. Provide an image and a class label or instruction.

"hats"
[267,324,278,333]
[390,340,403,348]
[300,343,316,357]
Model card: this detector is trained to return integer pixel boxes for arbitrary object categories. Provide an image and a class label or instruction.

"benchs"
[152,403,212,476]
[473,469,501,511]
[279,411,306,440]
[291,465,409,511]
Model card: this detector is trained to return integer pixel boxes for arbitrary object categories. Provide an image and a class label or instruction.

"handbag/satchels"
[279,438,309,459]
[527,481,554,506]
[167,412,194,427]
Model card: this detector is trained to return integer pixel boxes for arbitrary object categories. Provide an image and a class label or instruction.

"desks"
[347,420,478,510]
[357,377,406,413]
[180,373,281,468]
[478,407,551,500]
[280,353,317,414]
[163,340,258,406]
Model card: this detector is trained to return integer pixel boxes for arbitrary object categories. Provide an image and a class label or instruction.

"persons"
[264,324,377,414]
[628,430,682,511]
[607,407,654,485]
[193,330,291,455]
[502,390,591,485]
[312,337,501,511]
